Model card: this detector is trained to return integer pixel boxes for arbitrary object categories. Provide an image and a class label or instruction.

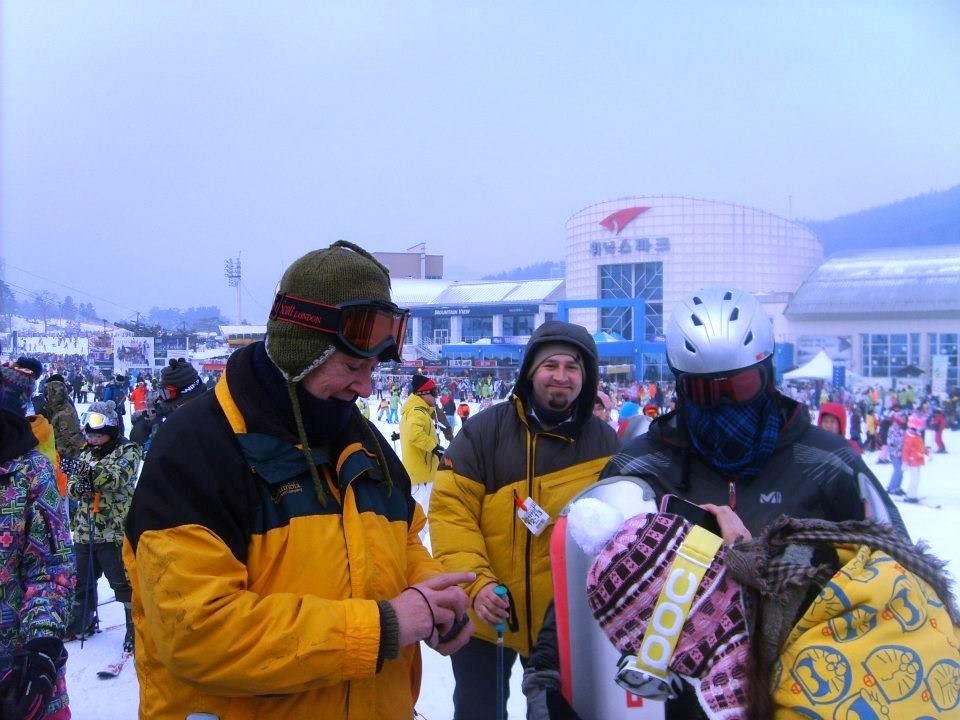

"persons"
[0,241,960,720]
[67,400,141,651]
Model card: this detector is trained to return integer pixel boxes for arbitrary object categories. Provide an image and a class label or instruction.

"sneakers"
[123,623,135,653]
[65,614,93,637]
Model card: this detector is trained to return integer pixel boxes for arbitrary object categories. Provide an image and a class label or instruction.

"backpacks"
[462,405,469,416]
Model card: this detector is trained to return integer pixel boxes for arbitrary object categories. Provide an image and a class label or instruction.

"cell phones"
[659,494,722,540]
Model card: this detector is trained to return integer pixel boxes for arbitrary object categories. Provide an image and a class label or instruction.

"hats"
[265,241,393,507]
[526,341,586,386]
[0,365,36,416]
[907,412,926,430]
[893,412,907,424]
[411,375,436,392]
[160,357,204,408]
[79,400,119,434]
[585,512,751,720]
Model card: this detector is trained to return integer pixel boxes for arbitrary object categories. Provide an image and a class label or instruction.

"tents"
[783,351,859,385]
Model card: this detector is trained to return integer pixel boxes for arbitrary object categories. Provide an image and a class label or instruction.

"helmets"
[617,414,654,445]
[664,286,776,373]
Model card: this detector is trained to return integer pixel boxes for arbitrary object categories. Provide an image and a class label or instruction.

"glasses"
[330,298,411,363]
[78,412,107,430]
[612,655,681,702]
[430,387,439,397]
[160,388,175,402]
[677,364,769,410]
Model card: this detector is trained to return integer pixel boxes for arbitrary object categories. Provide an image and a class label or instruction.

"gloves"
[431,444,445,461]
[525,689,581,720]
[0,636,68,720]
[391,431,400,441]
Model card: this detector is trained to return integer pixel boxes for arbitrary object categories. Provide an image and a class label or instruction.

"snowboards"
[550,475,665,720]
[98,595,116,608]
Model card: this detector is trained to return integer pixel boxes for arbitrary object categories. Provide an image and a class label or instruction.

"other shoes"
[904,498,919,503]
[887,488,906,496]
[937,447,946,453]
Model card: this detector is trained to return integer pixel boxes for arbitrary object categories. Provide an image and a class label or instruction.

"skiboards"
[63,623,134,677]
[898,495,941,510]
